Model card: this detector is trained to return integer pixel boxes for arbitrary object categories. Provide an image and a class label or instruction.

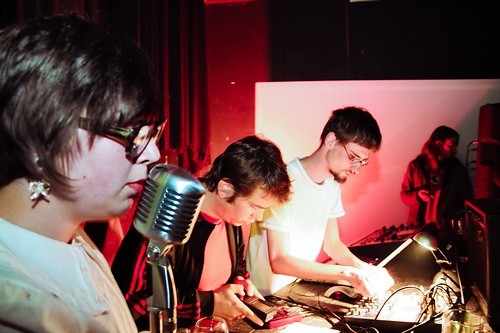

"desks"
[230,243,497,333]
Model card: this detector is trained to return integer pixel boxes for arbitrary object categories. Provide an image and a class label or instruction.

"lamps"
[414,232,466,333]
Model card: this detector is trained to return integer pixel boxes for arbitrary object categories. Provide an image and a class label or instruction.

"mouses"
[324,285,360,302]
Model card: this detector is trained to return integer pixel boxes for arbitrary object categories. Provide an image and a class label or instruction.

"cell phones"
[235,293,278,322]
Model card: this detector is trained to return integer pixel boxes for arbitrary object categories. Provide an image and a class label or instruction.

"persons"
[400,125,474,231]
[244,106,394,298]
[110,135,291,329]
[0,11,167,333]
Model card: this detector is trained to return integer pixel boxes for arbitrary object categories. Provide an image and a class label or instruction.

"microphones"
[133,164,206,309]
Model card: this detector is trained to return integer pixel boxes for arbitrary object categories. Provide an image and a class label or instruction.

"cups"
[442,310,484,333]
[193,316,229,333]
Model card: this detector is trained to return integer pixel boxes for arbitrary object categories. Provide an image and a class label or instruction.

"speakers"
[474,103,500,206]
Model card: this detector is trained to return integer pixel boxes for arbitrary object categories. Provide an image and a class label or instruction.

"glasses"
[343,143,369,166]
[79,116,167,164]
